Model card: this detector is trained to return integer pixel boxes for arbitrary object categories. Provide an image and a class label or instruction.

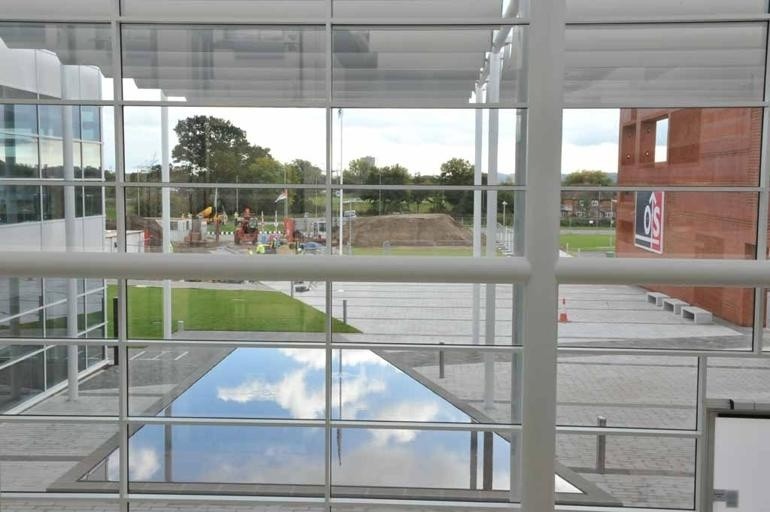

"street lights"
[501,200,508,250]
[610,199,617,250]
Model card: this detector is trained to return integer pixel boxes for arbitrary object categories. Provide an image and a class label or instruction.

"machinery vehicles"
[233,208,259,245]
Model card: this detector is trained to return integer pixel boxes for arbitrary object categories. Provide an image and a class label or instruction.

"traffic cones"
[558,298,570,322]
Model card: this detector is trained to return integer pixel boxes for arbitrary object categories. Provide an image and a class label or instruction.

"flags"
[275,189,288,202]
